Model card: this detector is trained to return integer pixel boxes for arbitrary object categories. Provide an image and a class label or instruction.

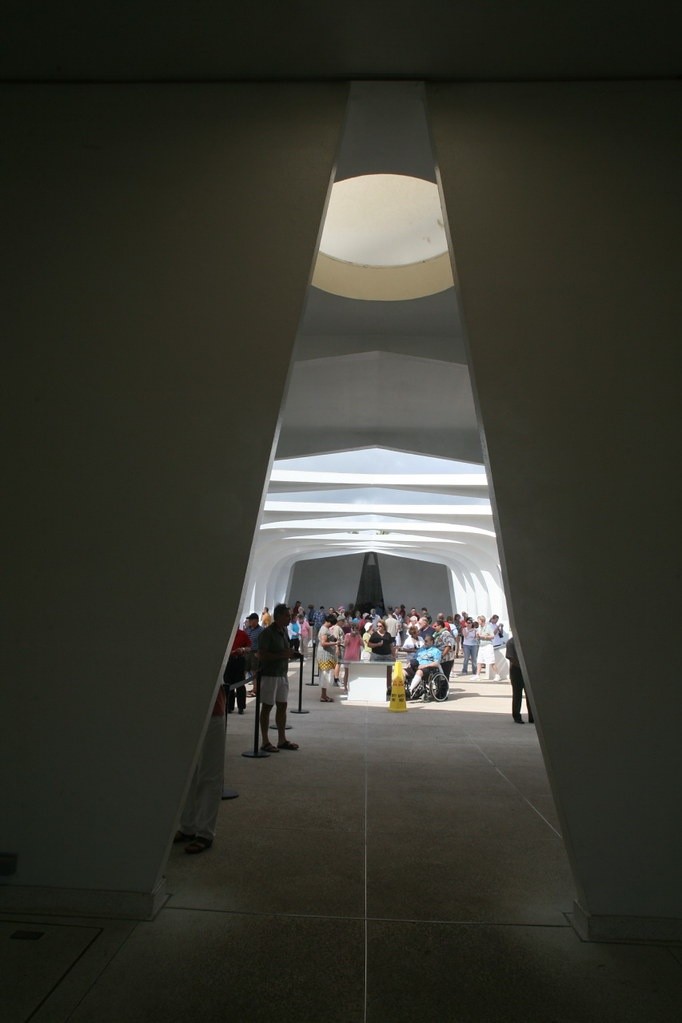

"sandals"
[320,696,334,702]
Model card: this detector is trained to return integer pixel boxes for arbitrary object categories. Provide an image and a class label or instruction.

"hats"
[246,613,258,619]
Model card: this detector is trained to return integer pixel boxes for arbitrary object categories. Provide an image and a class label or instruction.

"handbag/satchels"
[318,658,336,671]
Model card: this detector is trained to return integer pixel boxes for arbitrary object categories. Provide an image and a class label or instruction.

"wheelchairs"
[405,657,450,703]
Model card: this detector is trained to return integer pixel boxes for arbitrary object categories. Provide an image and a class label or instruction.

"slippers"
[261,742,279,752]
[277,741,299,750]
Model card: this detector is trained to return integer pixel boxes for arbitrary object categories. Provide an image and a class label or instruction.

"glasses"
[425,640,428,642]
[377,625,382,627]
[467,622,471,624]
[434,626,436,627]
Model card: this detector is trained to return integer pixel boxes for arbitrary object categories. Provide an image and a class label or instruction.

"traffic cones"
[387,662,408,713]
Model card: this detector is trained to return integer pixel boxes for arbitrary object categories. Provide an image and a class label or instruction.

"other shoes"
[238,708,244,714]
[228,709,232,713]
[529,720,534,723]
[185,836,213,853]
[470,676,481,681]
[173,830,196,843]
[515,718,524,723]
[333,680,342,686]
[493,674,501,682]
[450,673,458,678]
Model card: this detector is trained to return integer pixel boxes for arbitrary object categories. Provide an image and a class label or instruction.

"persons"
[505,635,535,724]
[242,613,265,697]
[173,685,227,855]
[226,625,252,715]
[260,607,272,627]
[286,601,510,702]
[256,605,300,754]
